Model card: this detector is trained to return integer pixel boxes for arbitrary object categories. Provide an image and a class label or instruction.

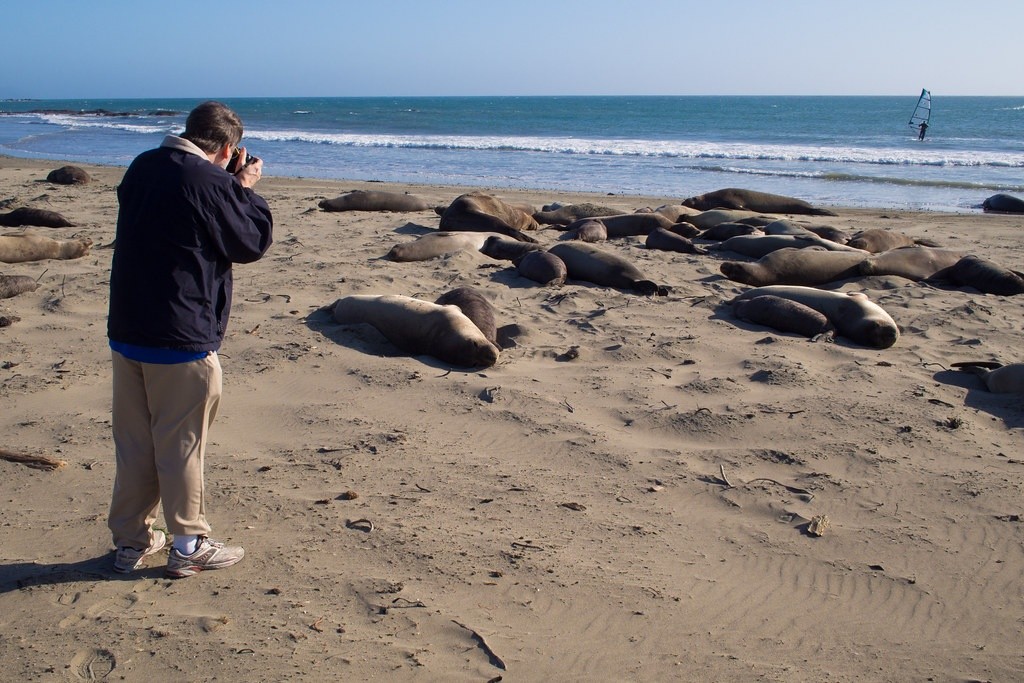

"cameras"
[225,148,254,172]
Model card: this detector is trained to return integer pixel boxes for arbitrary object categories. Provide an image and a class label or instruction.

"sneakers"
[114,530,166,574]
[165,535,244,577]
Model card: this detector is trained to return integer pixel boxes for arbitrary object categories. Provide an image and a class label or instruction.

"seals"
[0,166,95,299]
[317,182,1024,398]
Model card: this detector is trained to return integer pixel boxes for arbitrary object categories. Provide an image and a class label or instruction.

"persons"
[109,101,274,574]
[918,121,929,141]
